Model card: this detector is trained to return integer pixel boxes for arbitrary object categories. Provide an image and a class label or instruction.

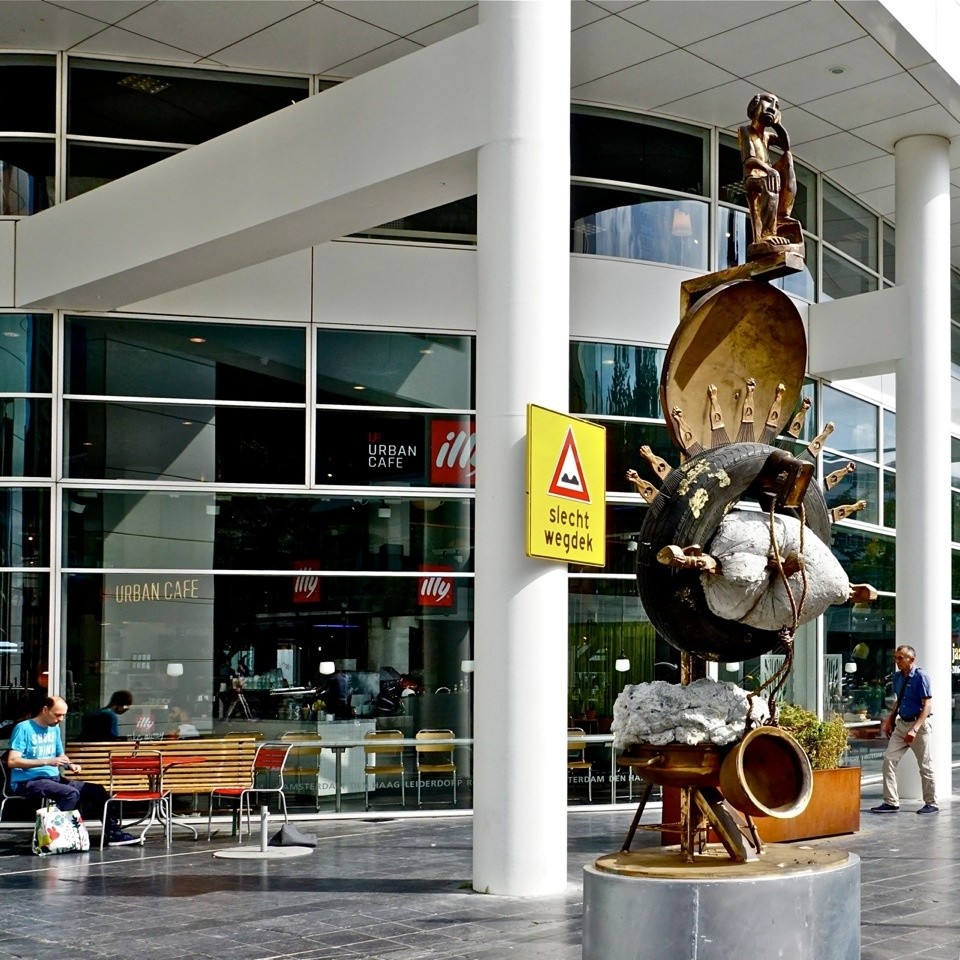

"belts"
[901,713,932,722]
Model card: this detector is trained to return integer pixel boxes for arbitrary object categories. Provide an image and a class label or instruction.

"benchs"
[61,738,258,837]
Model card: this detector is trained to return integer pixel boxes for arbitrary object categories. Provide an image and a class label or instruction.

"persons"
[81,690,133,738]
[871,645,939,813]
[8,697,146,846]
[158,700,191,740]
[738,93,797,245]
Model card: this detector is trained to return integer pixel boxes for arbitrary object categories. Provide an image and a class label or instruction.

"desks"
[845,719,881,730]
[121,757,205,845]
[259,738,472,812]
[567,734,617,805]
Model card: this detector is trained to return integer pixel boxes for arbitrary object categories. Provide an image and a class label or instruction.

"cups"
[277,711,286,720]
[326,714,334,722]
[309,710,317,721]
[301,707,309,720]
[232,668,282,690]
[318,710,324,721]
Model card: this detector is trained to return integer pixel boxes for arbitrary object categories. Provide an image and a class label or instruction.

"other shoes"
[916,805,939,814]
[103,829,145,846]
[871,803,900,813]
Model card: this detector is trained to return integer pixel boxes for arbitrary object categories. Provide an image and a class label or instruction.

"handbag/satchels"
[31,806,90,857]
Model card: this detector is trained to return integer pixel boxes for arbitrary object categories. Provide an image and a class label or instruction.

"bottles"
[286,703,294,720]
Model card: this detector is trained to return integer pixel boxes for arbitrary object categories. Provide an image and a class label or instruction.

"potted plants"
[742,704,862,844]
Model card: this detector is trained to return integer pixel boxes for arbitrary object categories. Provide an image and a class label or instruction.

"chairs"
[568,727,662,801]
[362,729,407,811]
[0,727,324,852]
[416,726,457,807]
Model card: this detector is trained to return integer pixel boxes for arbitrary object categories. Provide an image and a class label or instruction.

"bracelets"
[913,728,919,733]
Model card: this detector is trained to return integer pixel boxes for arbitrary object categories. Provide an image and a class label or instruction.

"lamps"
[671,208,693,265]
[845,604,857,673]
[461,660,473,672]
[167,662,183,678]
[320,612,335,675]
[615,596,630,672]
[725,661,740,672]
